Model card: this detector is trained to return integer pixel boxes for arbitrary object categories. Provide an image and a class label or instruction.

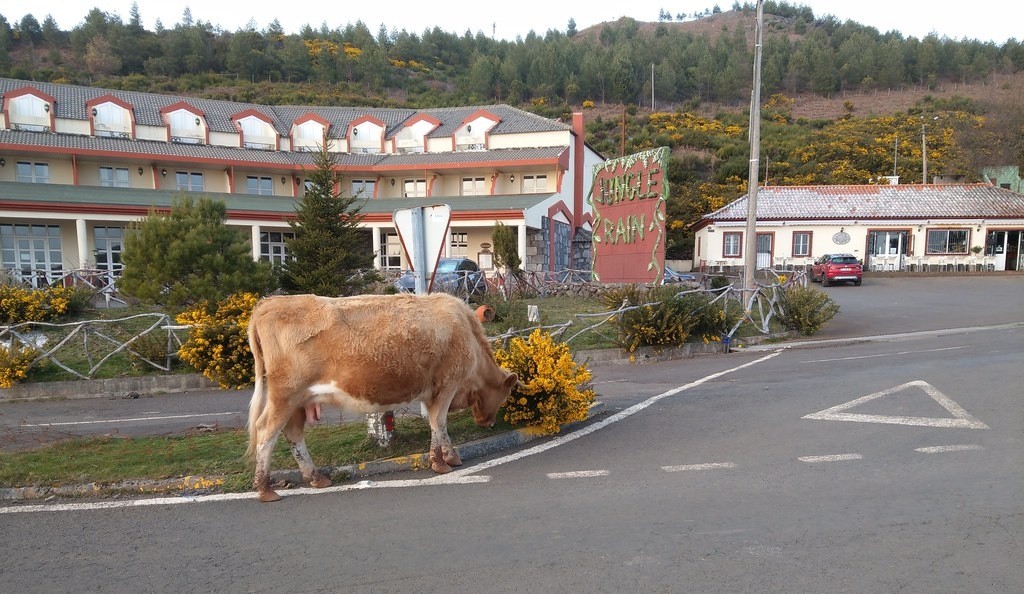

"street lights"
[919,114,940,184]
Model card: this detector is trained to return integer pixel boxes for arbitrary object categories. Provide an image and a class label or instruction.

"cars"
[392,256,486,303]
[809,253,862,287]
[664,266,696,285]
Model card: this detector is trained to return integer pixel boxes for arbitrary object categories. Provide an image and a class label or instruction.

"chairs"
[868,256,997,273]
[774,257,796,272]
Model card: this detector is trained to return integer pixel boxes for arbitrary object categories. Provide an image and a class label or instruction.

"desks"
[715,260,726,272]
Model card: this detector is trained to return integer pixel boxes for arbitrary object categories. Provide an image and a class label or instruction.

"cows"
[240,290,518,503]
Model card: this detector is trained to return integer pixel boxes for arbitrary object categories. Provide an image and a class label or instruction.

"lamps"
[510,175,514,183]
[195,117,200,126]
[162,169,167,177]
[44,103,49,112]
[918,225,922,232]
[138,166,143,176]
[390,178,395,186]
[92,108,97,117]
[0,157,5,167]
[93,249,97,256]
[976,226,981,231]
[353,128,357,135]
[296,177,300,186]
[841,227,844,232]
[467,125,471,132]
[281,177,285,185]
